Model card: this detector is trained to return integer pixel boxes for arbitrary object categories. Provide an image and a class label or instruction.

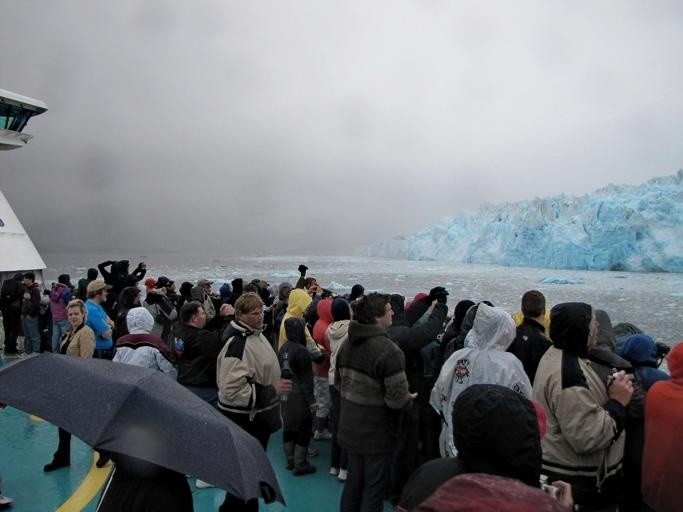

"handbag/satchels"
[270,306,275,329]
[280,343,301,394]
[154,291,169,324]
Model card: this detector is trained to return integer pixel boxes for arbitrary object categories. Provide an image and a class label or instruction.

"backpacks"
[1,279,17,304]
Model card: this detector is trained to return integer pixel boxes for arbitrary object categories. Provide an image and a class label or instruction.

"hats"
[86,279,113,292]
[155,276,169,286]
[144,277,157,287]
[197,278,214,287]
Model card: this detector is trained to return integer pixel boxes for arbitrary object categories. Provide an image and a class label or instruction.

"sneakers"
[27,352,40,358]
[329,467,338,476]
[15,352,27,358]
[0,494,15,506]
[337,467,349,481]
[194,478,217,488]
[308,446,319,455]
[313,429,334,440]
[185,472,193,479]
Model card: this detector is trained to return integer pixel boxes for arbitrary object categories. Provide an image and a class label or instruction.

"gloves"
[436,294,447,304]
[297,264,308,275]
[428,286,449,300]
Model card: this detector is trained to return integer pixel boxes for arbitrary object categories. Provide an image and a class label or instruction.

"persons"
[587,309,645,417]
[278,317,319,475]
[218,278,259,294]
[143,287,177,342]
[273,286,323,349]
[95,471,193,512]
[273,282,293,306]
[294,264,308,289]
[505,290,553,385]
[44,299,110,473]
[405,292,428,309]
[325,286,348,298]
[157,276,169,286]
[115,287,141,335]
[415,473,534,512]
[306,277,317,292]
[641,341,683,512]
[0,311,5,350]
[216,292,293,512]
[84,280,114,360]
[397,384,573,511]
[312,299,335,439]
[279,288,330,457]
[180,281,194,303]
[51,282,56,288]
[325,300,354,481]
[172,301,234,488]
[611,322,642,342]
[0,492,12,507]
[20,273,41,357]
[430,302,533,456]
[533,302,635,511]
[86,269,98,282]
[166,281,181,302]
[444,305,478,359]
[70,283,76,291]
[350,285,364,300]
[112,307,177,380]
[145,277,157,293]
[258,280,270,298]
[389,294,405,325]
[616,334,671,392]
[334,293,418,512]
[119,260,146,286]
[42,288,51,351]
[77,279,86,298]
[197,279,216,329]
[478,299,493,305]
[98,261,122,295]
[191,287,204,303]
[440,299,475,357]
[51,274,74,353]
[1,273,24,354]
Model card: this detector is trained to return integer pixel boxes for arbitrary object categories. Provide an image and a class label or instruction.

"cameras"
[652,340,671,360]
[435,286,448,297]
[604,371,636,390]
[298,264,308,273]
[540,480,566,504]
[139,262,146,268]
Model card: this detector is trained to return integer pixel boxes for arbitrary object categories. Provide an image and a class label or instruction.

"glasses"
[165,284,170,287]
[136,294,140,298]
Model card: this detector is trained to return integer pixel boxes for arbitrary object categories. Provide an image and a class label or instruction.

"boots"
[292,444,317,476]
[282,441,294,470]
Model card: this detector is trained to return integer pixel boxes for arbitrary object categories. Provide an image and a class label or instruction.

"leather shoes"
[96,452,112,468]
[44,458,70,471]
[3,351,22,355]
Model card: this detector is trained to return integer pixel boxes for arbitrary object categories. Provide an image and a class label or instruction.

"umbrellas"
[0,188,47,272]
[1,355,286,511]
